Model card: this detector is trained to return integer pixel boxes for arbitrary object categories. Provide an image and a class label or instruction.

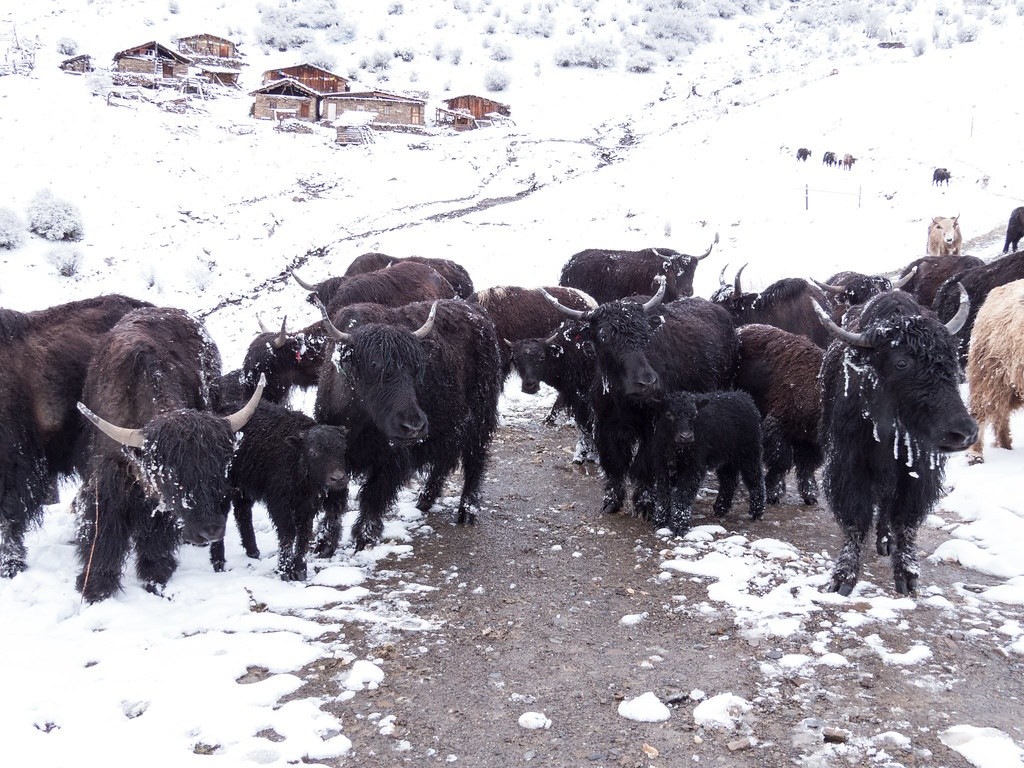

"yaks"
[1,207,1024,602]
